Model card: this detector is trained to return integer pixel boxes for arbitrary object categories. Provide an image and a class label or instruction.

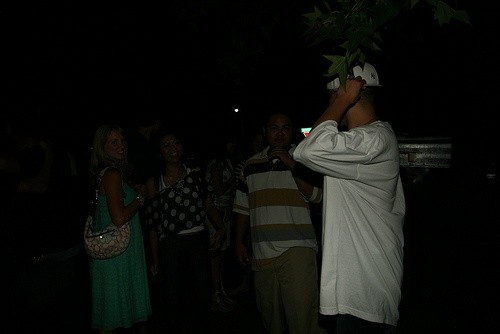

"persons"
[292,62,406,334]
[144,130,226,334]
[204,128,254,334]
[231,108,322,334]
[87,124,152,334]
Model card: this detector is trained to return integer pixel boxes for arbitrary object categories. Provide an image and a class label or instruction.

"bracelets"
[137,195,144,206]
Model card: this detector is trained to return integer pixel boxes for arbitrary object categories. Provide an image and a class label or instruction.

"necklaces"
[167,176,185,196]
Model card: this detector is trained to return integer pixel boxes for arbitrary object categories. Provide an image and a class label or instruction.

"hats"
[327,61,383,89]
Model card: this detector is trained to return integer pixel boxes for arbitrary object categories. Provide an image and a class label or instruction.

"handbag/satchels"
[83,211,131,260]
